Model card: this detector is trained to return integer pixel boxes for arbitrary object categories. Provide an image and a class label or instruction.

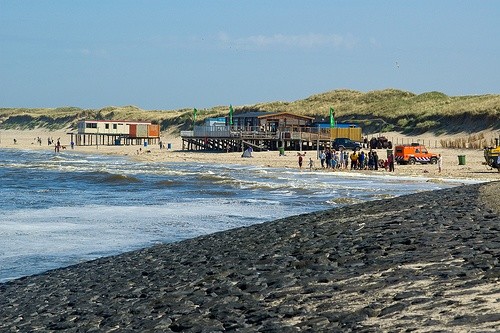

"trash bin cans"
[116,140,120,145]
[458,155,465,165]
[280,147,284,154]
[168,144,171,148]
[144,142,147,146]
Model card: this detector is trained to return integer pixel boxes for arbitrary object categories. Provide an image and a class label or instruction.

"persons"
[437,154,442,172]
[298,149,396,171]
[13,135,74,152]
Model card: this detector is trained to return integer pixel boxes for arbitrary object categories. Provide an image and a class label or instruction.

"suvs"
[394,145,439,165]
[332,138,362,152]
